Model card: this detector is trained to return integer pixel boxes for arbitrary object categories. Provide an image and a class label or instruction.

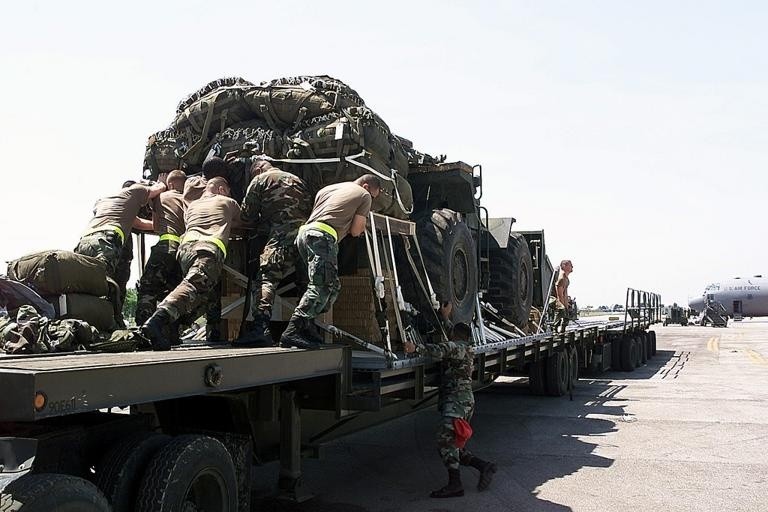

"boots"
[431,465,465,498]
[278,314,325,350]
[170,320,183,344]
[208,323,220,342]
[142,308,171,350]
[468,456,497,491]
[231,315,273,349]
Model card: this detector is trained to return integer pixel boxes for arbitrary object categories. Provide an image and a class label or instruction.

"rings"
[406,347,409,350]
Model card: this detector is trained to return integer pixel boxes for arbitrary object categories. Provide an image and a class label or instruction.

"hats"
[454,418,473,448]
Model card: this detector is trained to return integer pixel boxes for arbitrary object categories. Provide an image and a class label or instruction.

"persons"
[404,299,498,499]
[181,151,239,233]
[231,160,314,348]
[135,170,188,326]
[554,260,573,327]
[278,175,381,350]
[139,178,244,351]
[74,172,167,308]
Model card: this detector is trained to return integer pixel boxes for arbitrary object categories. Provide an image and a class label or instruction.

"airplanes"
[688,274,767,323]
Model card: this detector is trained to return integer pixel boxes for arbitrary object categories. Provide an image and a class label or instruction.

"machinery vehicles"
[662,302,699,326]
